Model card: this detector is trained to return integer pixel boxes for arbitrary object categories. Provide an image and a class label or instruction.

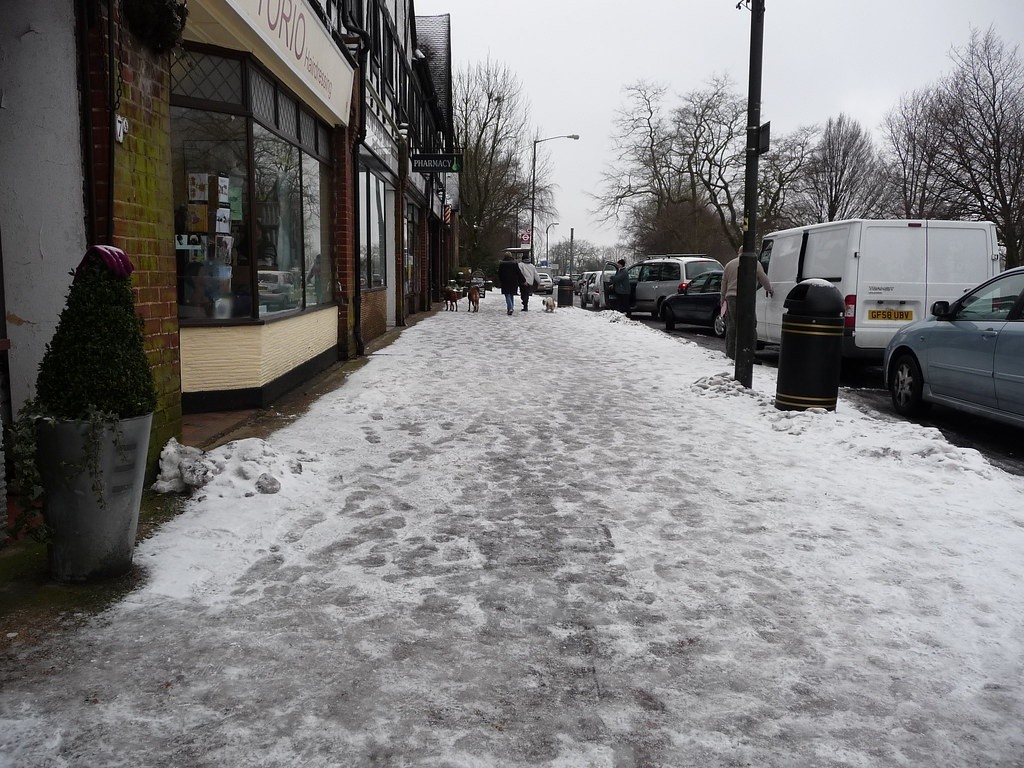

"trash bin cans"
[557,276,574,308]
[775,278,846,413]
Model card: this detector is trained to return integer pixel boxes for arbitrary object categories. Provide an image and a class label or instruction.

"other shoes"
[508,310,513,315]
[752,359,762,365]
[522,309,528,311]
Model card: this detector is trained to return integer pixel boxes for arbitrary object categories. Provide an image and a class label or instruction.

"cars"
[659,270,727,338]
[553,271,632,309]
[883,267,1024,428]
[533,272,554,295]
[258,271,303,312]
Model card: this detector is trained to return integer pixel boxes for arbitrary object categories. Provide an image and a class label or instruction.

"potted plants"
[0,248,159,586]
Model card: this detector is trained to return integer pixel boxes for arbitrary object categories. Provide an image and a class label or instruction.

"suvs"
[600,254,725,320]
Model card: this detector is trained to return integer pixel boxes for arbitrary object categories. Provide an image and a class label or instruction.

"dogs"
[542,297,556,312]
[440,286,458,311]
[468,286,480,313]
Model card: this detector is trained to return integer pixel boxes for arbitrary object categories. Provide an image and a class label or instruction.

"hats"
[618,259,625,267]
[739,245,743,253]
[522,255,529,260]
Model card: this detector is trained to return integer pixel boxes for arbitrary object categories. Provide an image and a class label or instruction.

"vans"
[756,218,1001,382]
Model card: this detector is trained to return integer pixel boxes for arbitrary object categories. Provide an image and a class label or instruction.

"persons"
[235,218,276,266]
[499,252,526,315]
[307,253,322,293]
[518,255,541,311]
[610,260,631,319]
[721,245,773,359]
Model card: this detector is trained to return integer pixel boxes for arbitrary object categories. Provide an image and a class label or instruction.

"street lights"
[531,134,579,265]
[546,222,559,268]
